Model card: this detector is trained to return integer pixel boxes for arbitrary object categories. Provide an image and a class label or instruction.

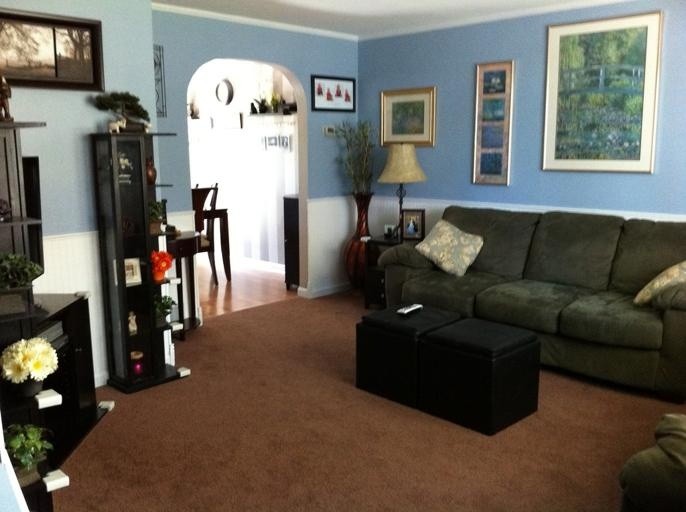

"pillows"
[633,261,686,312]
[415,221,483,276]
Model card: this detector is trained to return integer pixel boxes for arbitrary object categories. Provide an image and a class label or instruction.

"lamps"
[377,142,425,240]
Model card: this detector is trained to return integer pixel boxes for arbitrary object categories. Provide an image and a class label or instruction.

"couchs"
[377,206,686,403]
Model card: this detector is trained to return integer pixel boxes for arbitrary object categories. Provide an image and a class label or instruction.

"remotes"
[396,304,424,315]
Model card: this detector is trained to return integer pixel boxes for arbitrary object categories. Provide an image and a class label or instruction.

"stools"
[419,318,541,436]
[354,305,462,405]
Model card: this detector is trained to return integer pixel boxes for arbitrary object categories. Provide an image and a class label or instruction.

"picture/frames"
[400,208,425,239]
[380,85,437,148]
[541,9,665,175]
[1,8,106,91]
[310,76,358,113]
[473,60,514,184]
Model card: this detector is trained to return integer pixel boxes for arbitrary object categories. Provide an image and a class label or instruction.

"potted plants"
[153,294,177,329]
[4,424,54,477]
[334,118,378,289]
[148,199,164,235]
[89,90,151,132]
[1,252,42,318]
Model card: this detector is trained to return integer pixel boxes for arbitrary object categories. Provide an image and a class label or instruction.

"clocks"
[216,81,232,106]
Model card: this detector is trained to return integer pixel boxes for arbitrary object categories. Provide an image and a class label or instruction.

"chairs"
[190,185,232,283]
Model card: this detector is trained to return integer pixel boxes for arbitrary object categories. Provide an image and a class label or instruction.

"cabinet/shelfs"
[284,195,301,291]
[1,77,69,512]
[88,130,192,394]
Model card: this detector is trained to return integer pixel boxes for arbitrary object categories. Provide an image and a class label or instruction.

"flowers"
[3,338,58,383]
[151,249,174,273]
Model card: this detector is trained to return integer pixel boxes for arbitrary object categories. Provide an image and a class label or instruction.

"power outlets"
[324,126,336,136]
[384,224,397,236]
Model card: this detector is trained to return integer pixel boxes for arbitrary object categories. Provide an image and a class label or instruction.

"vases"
[154,270,166,281]
[19,377,44,397]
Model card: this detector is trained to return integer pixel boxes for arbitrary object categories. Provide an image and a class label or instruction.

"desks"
[34,292,110,472]
[165,230,202,336]
